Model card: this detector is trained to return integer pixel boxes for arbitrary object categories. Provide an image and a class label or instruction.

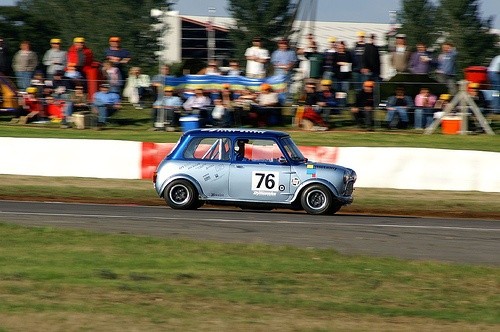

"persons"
[11,31,500,133]
[225,138,249,162]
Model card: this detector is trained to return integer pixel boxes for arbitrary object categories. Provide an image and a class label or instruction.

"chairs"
[222,140,230,160]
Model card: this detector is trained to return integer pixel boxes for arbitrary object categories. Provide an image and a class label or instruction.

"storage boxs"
[440,117,464,135]
[178,114,206,132]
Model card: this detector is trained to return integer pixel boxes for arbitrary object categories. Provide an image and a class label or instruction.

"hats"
[328,37,337,42]
[396,34,407,38]
[74,37,85,43]
[164,87,176,92]
[27,87,38,93]
[306,78,317,84]
[50,38,63,44]
[260,85,271,90]
[440,94,450,99]
[110,37,121,42]
[321,80,333,86]
[468,82,479,88]
[358,32,365,37]
[100,83,111,88]
[364,81,374,87]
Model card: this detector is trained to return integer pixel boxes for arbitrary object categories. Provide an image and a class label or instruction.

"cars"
[153,127,357,215]
